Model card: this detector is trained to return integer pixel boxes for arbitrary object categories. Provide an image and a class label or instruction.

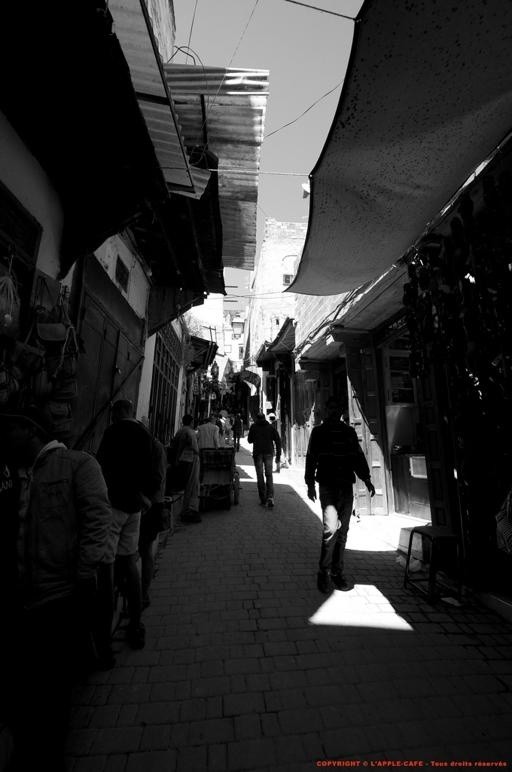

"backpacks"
[115,416,167,491]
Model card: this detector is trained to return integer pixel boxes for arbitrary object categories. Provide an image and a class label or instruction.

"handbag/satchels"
[0,272,79,414]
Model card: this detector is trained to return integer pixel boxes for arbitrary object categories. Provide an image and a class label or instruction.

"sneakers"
[315,569,348,593]
[118,598,153,647]
[262,496,275,508]
[182,511,202,525]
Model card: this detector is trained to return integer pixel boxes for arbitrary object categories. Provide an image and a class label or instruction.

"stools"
[405,526,463,609]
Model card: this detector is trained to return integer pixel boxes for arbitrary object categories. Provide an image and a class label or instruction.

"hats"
[1,403,51,438]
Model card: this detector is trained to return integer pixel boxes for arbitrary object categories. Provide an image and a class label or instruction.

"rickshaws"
[199,447,242,510]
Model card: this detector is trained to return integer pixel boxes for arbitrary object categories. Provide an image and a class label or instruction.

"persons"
[95,399,162,672]
[132,421,168,605]
[195,402,244,452]
[304,396,375,593]
[0,405,115,772]
[174,414,199,492]
[248,412,281,510]
